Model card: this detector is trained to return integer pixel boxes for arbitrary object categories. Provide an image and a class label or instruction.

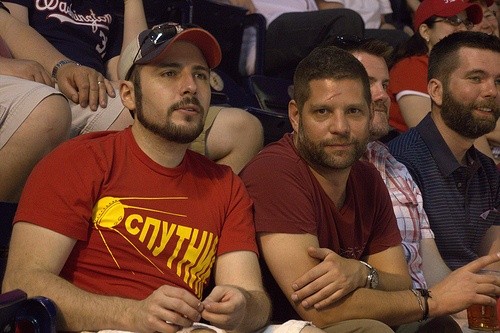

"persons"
[335,36,471,333]
[0,21,326,333]
[386,30,500,271]
[0,0,500,190]
[202,44,500,333]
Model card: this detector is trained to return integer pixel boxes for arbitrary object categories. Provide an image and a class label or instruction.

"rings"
[98,81,105,84]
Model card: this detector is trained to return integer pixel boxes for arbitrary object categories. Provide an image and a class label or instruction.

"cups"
[467,270,500,331]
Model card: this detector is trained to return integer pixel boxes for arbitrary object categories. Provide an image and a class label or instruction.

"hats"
[412,0,483,34]
[117,21,222,84]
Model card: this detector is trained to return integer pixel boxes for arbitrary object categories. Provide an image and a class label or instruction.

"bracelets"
[416,289,429,320]
[52,59,75,79]
[411,289,425,318]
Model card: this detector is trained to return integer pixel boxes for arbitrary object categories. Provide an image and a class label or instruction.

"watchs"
[360,260,379,288]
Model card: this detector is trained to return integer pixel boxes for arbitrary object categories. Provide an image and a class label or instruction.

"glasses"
[427,15,475,27]
[132,22,201,62]
